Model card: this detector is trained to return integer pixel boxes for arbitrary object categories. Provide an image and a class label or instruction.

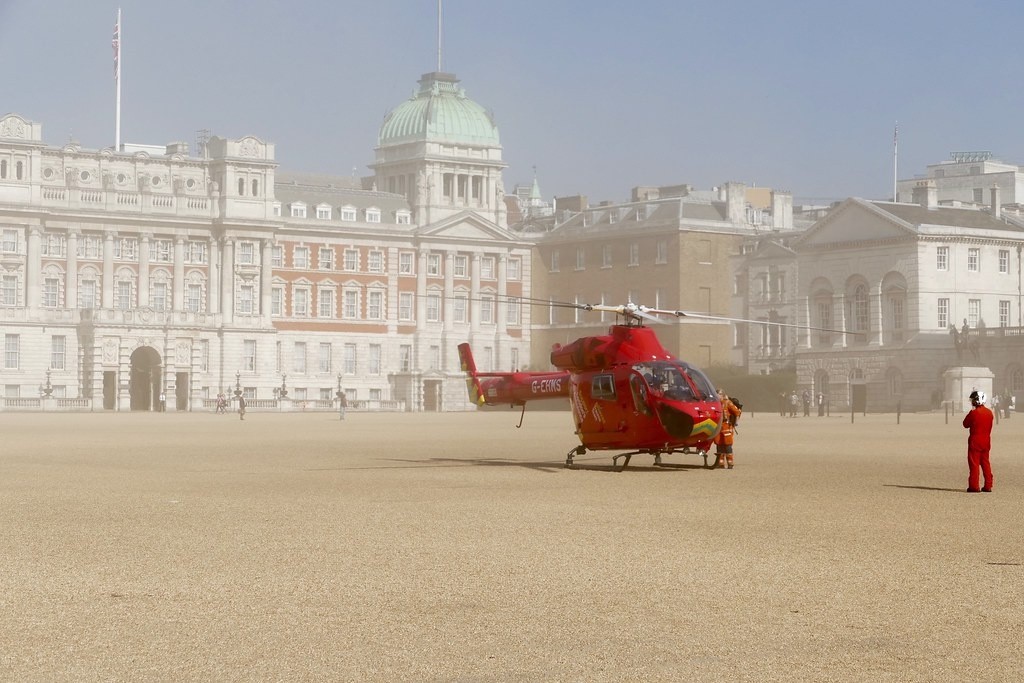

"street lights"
[231,371,243,402]
[279,373,289,401]
[334,373,343,402]
[42,367,54,397]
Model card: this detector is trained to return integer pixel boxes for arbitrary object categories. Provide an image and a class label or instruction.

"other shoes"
[713,464,734,469]
[967,487,993,492]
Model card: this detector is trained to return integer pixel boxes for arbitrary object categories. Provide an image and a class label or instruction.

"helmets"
[715,389,725,394]
[969,391,986,405]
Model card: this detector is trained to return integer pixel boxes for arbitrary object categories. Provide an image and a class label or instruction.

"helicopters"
[454,289,870,473]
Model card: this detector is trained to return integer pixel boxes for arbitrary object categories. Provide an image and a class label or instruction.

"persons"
[715,388,740,469]
[778,391,786,416]
[789,390,798,418]
[644,373,666,410]
[802,388,811,417]
[961,318,970,349]
[240,393,247,420]
[339,394,349,420]
[158,391,166,413]
[216,393,228,414]
[961,390,994,493]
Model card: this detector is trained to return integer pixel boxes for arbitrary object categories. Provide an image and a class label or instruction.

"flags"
[112,17,120,84]
